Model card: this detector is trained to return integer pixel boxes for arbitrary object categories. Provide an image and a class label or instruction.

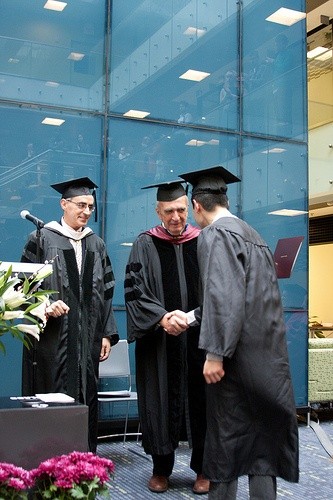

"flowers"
[0,450,117,500]
[0,252,60,362]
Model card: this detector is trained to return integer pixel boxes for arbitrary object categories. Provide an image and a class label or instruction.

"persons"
[124,179,210,494]
[164,166,299,500]
[14,177,120,453]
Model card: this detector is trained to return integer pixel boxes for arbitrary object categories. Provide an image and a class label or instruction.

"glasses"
[67,200,95,212]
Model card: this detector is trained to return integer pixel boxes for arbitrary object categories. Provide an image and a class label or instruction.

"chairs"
[95,339,140,451]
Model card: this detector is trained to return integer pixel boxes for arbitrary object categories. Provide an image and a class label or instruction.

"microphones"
[20,210,45,228]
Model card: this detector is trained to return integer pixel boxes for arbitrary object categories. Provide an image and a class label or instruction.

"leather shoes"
[193,473,210,494]
[148,475,170,491]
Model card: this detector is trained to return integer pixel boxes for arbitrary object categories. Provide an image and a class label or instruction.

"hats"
[178,166,241,196]
[141,180,190,201]
[50,177,99,223]
